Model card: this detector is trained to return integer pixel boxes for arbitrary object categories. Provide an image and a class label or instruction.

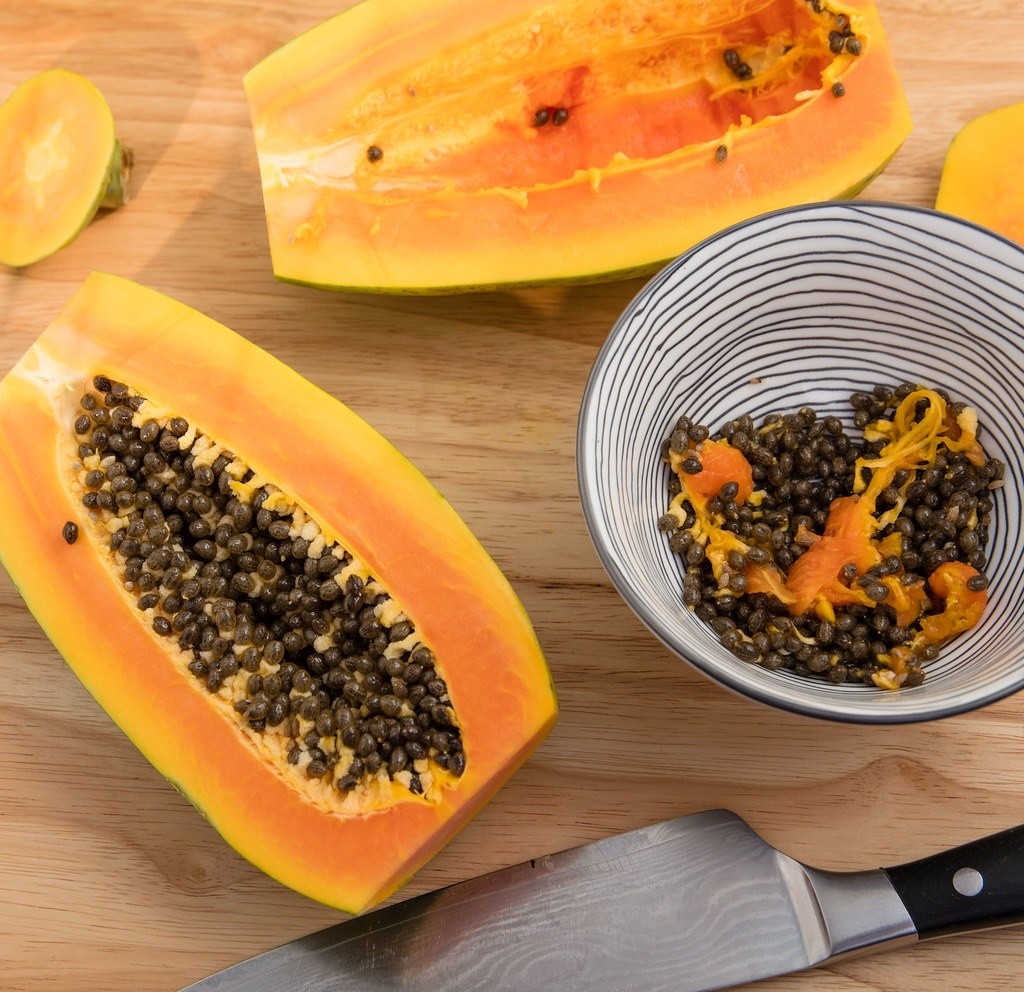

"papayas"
[936,102,1024,260]
[0,269,558,920]
[243,1,918,301]
[1,67,135,267]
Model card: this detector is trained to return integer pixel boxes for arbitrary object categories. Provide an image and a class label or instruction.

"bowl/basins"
[575,201,1024,725]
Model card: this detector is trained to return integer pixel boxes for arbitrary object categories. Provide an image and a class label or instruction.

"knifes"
[182,810,1023,991]
